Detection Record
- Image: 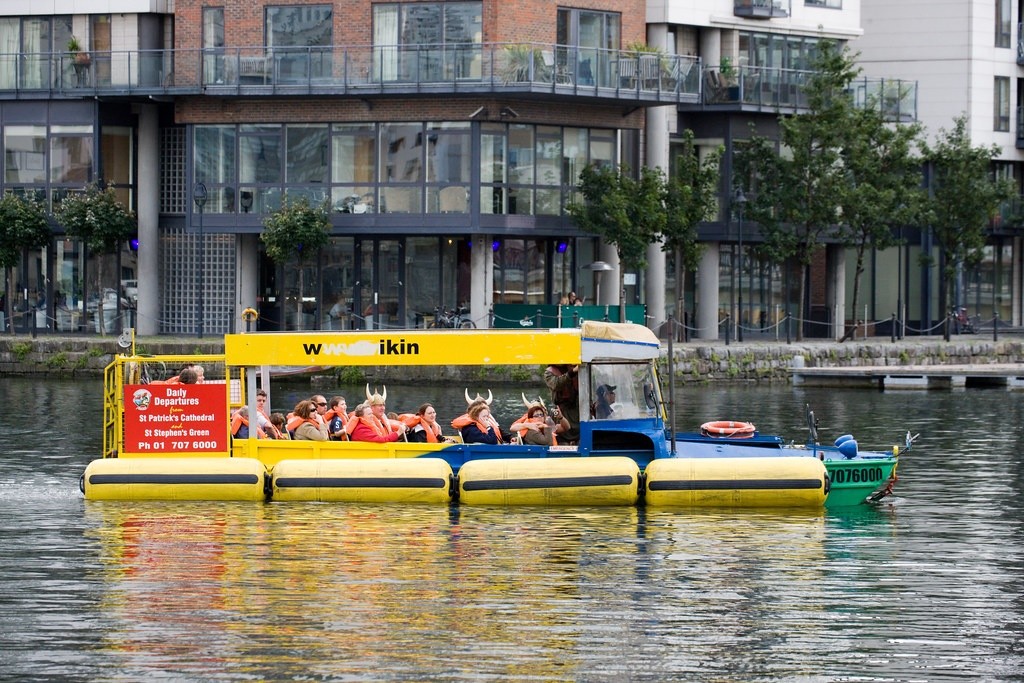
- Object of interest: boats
[79,309,923,515]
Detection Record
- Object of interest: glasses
[532,414,544,417]
[310,408,316,412]
[317,403,327,406]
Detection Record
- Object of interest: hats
[596,384,617,397]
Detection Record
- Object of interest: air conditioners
[224,55,272,77]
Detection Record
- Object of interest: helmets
[521,392,546,412]
[366,383,387,405]
[465,388,493,406]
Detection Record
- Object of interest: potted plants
[622,41,670,89]
[498,44,546,83]
[65,36,88,64]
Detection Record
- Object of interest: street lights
[732,189,749,343]
[580,261,615,306]
[192,182,207,339]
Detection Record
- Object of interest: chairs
[401,423,410,443]
[541,50,573,84]
[617,55,658,89]
[343,426,352,442]
[284,425,292,440]
[704,70,757,101]
[662,60,695,93]
[516,431,524,446]
[457,429,465,444]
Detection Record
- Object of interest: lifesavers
[701,420,755,433]
[700,432,755,439]
[241,308,258,322]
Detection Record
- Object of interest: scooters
[947,305,983,335]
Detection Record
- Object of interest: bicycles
[426,305,477,330]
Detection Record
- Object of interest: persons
[287,394,350,441]
[232,389,285,440]
[593,384,617,419]
[452,398,570,446]
[545,364,579,442]
[363,298,385,316]
[559,291,582,306]
[166,361,204,384]
[330,297,346,328]
[347,394,454,444]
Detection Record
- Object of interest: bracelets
[559,417,563,420]
